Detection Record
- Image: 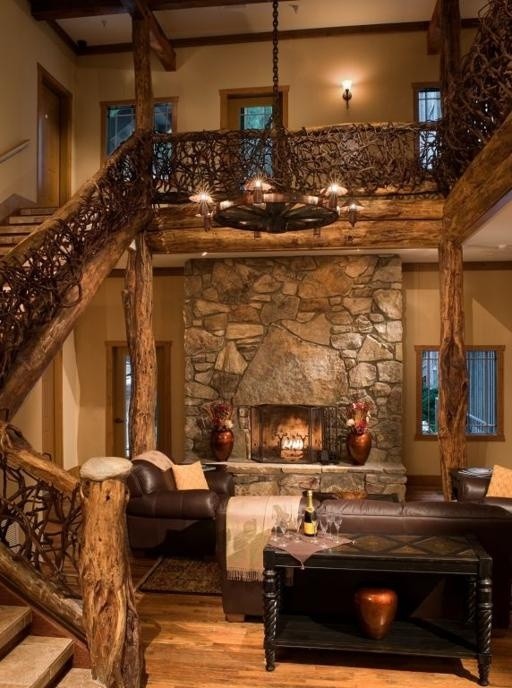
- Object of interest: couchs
[126,450,236,561]
[218,497,511,639]
[458,464,511,511]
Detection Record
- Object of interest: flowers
[204,404,234,431]
[343,401,371,434]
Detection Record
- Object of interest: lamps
[187,1,364,240]
[341,80,352,108]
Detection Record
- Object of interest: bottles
[302,489,319,537]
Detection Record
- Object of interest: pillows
[171,460,209,490]
[484,464,511,497]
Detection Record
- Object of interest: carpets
[133,558,225,596]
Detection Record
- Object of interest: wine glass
[310,509,343,546]
[267,510,306,548]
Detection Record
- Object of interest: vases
[346,433,372,466]
[210,430,235,462]
